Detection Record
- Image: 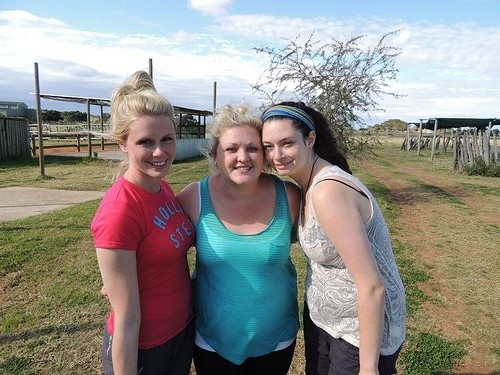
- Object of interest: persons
[258,101,410,375]
[88,67,200,374]
[99,101,305,375]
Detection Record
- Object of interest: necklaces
[299,154,319,228]
